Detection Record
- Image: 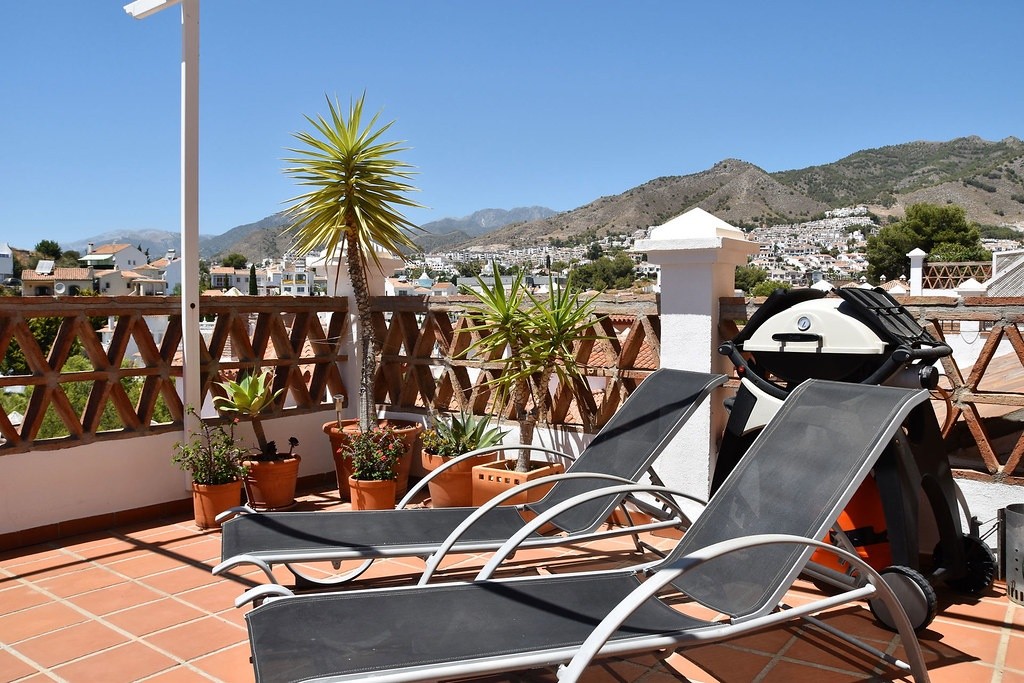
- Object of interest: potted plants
[210,364,301,508]
[420,408,511,507]
[169,402,246,528]
[446,257,617,533]
[278,89,425,500]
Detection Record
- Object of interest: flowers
[335,414,408,481]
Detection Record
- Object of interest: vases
[348,474,396,510]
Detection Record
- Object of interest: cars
[2,277,19,286]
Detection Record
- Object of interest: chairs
[212,368,730,600]
[247,376,926,683]
